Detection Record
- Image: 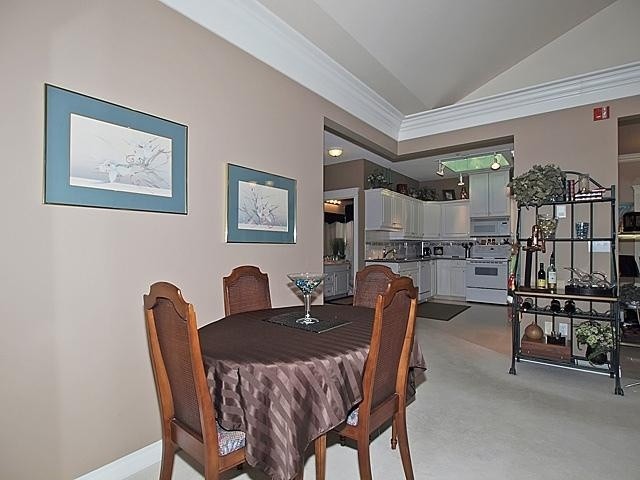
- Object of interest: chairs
[353,265,400,308]
[143,282,246,480]
[314,277,419,480]
[223,266,272,317]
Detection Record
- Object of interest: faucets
[383,248,396,258]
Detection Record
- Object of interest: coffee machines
[417,242,432,259]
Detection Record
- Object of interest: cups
[536,214,558,239]
[577,222,589,239]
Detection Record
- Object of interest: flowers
[575,322,616,359]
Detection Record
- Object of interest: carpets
[416,301,471,321]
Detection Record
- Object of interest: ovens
[464,260,509,305]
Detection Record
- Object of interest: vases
[586,344,607,365]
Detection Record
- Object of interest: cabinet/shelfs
[619,153,640,347]
[324,272,334,297]
[509,171,624,396]
[365,171,511,304]
[334,271,349,295]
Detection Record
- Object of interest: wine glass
[284,273,331,324]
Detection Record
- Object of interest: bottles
[551,300,561,315]
[522,297,533,312]
[546,256,557,289]
[465,245,470,258]
[564,302,576,317]
[536,262,546,290]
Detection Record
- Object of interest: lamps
[436,160,444,176]
[328,147,343,157]
[491,152,501,170]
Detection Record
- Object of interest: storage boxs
[521,334,571,363]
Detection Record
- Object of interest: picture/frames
[225,163,297,244]
[44,84,188,215]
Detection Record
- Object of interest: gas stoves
[465,256,509,262]
